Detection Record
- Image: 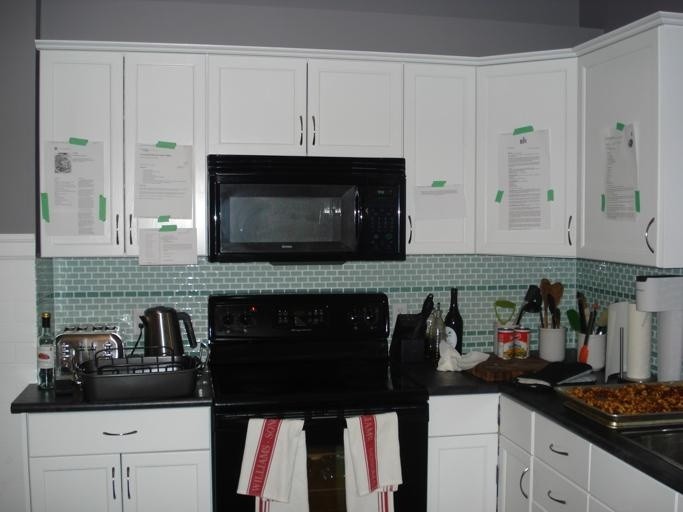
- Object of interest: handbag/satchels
[390,313,432,391]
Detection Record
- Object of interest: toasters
[53,325,126,374]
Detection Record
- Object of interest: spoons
[515,302,543,328]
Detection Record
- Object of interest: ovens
[213,403,427,512]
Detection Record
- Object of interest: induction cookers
[208,343,400,403]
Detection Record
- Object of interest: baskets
[95,344,183,375]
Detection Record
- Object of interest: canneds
[514,327,531,361]
[498,327,514,360]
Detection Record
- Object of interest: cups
[577,332,606,373]
[538,324,567,363]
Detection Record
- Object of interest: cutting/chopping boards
[469,357,548,382]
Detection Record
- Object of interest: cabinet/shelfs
[26,406,212,512]
[427,392,500,512]
[574,11,681,269]
[533,409,590,512]
[588,443,682,512]
[206,43,405,159]
[402,52,475,256]
[477,48,575,256]
[34,37,206,258]
[497,394,533,512]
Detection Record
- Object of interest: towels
[235,416,310,512]
[343,411,403,512]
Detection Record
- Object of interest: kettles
[138,306,198,356]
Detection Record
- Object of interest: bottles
[36,312,57,389]
[429,309,447,364]
[444,287,465,357]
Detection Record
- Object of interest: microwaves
[206,153,407,265]
